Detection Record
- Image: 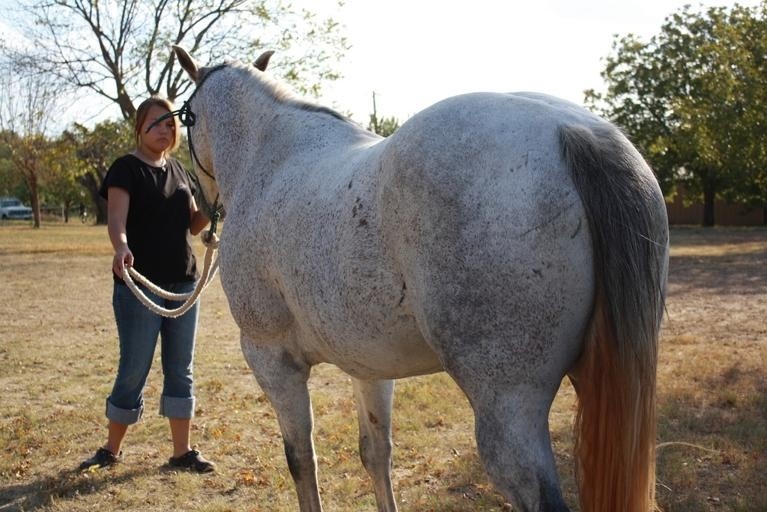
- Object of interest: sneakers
[168,444,216,472]
[80,447,122,470]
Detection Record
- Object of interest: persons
[78,97,222,474]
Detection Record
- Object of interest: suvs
[0,197,33,222]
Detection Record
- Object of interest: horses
[170,43,670,512]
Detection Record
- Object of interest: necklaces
[136,151,167,172]
